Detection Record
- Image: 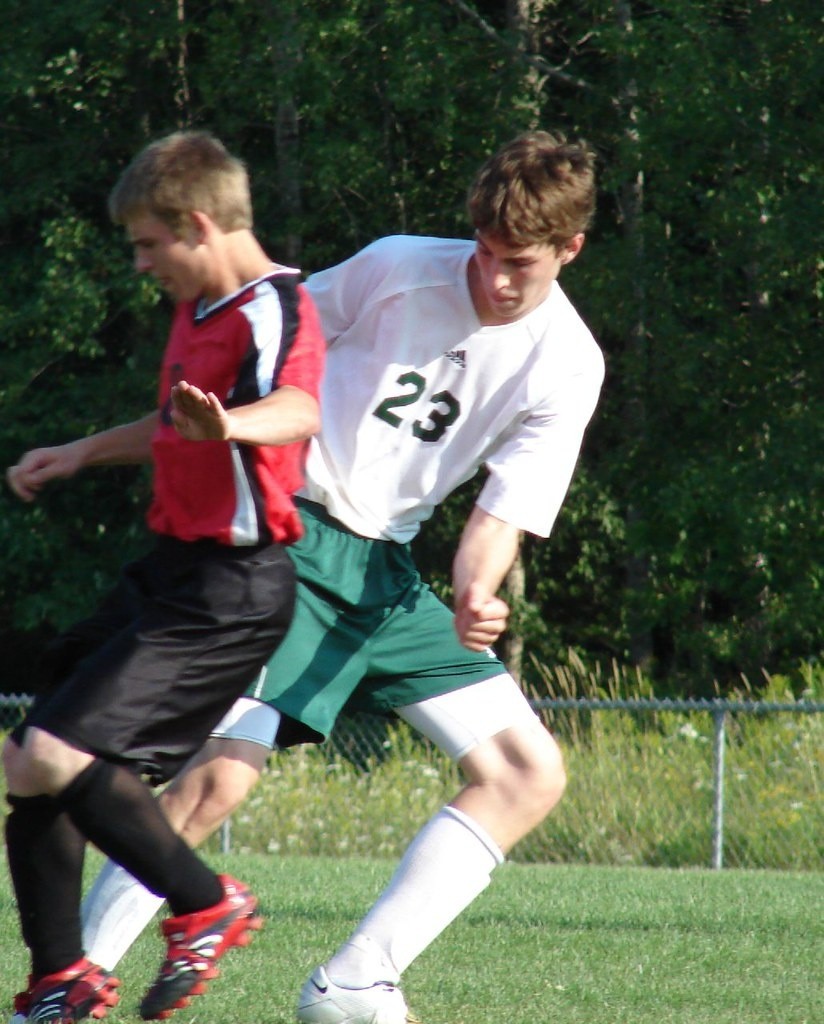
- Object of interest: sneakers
[12,957,121,1024]
[137,874,264,1020]
[297,965,420,1024]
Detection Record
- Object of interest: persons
[83,129,607,1023]
[3,130,324,1024]
